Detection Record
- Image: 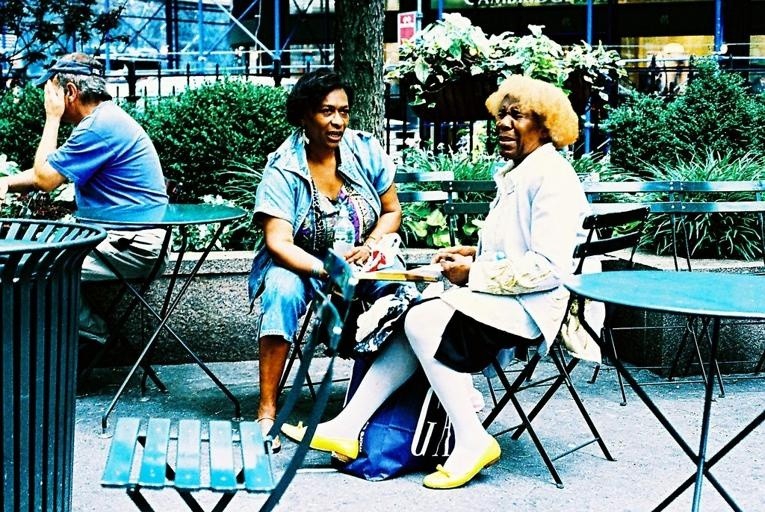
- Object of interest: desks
[562,269,765,512]
[71,202,249,439]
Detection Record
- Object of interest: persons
[0,52,173,389]
[281,73,610,490]
[247,69,403,455]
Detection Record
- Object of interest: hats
[31,60,105,89]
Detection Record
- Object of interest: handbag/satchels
[331,339,455,481]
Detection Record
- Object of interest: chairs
[100,249,344,510]
[47,180,184,395]
[439,178,498,242]
[581,180,715,407]
[394,168,454,266]
[675,179,765,394]
[484,207,649,487]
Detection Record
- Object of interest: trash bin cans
[0,217,107,512]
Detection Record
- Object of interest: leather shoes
[423,435,502,489]
[280,421,360,464]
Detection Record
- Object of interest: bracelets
[310,256,324,277]
[363,241,373,252]
